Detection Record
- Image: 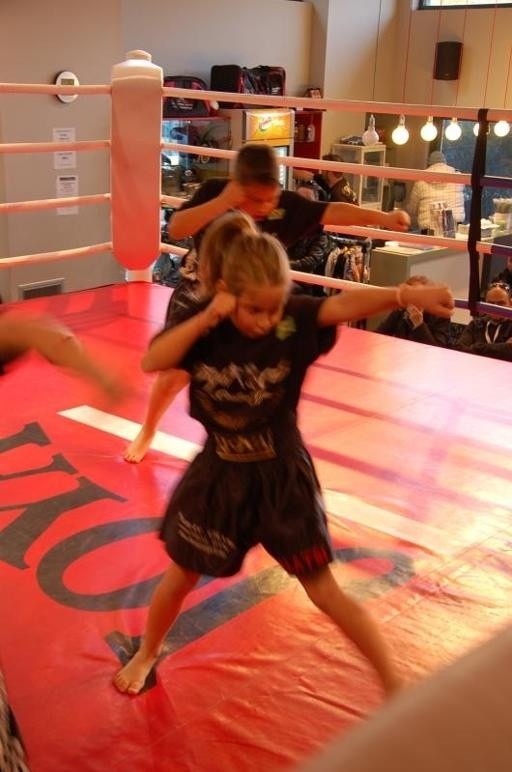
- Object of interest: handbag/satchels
[210,64,286,110]
[163,75,212,118]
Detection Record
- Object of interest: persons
[113,208,454,705]
[121,143,412,462]
[0,303,128,401]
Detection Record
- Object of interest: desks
[363,229,472,333]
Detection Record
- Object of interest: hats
[429,152,446,165]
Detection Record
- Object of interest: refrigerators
[217,107,295,193]
